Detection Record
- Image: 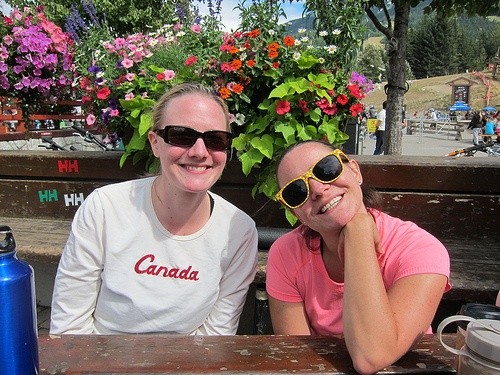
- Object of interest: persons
[358,100,500,155]
[50,84,259,335]
[266,139,452,375]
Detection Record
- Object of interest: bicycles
[443,140,500,157]
[38,126,123,150]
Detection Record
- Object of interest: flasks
[0,225,39,375]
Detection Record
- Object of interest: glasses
[153,124,234,152]
[274,148,350,212]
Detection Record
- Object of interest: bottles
[437,315,500,375]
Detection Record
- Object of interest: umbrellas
[482,106,496,115]
[448,101,471,114]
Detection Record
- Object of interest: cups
[453,303,500,372]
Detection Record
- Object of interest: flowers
[0,0,378,227]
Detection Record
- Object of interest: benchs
[478,133,500,143]
[0,149,500,337]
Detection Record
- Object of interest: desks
[35,333,465,375]
